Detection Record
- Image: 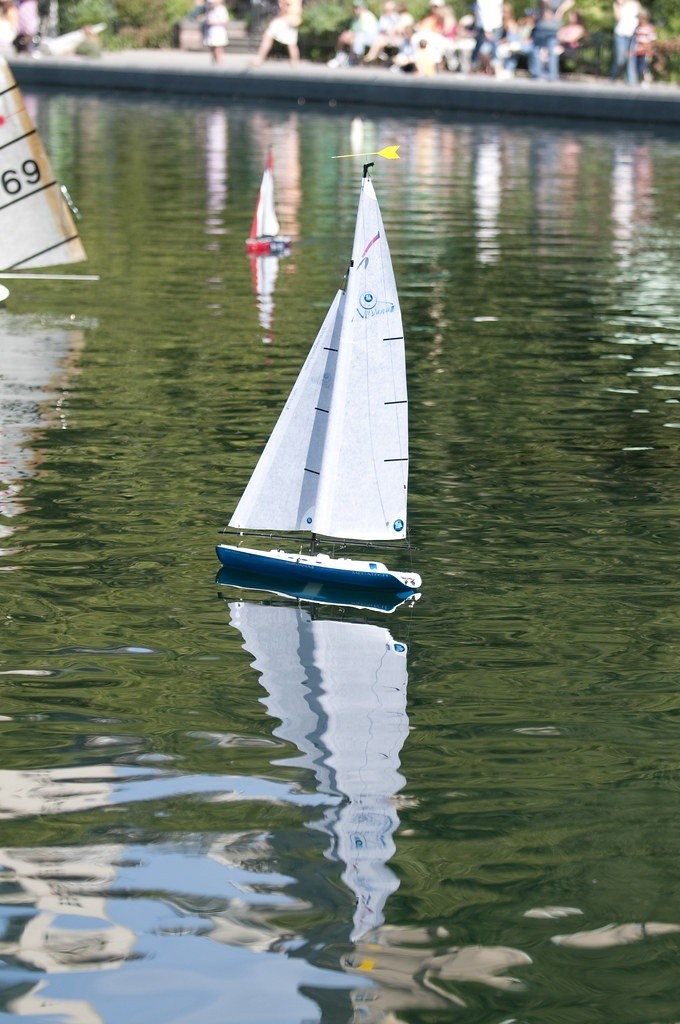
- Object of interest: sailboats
[0,53,100,303]
[244,146,291,255]
[208,566,426,977]
[208,143,424,593]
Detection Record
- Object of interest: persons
[0,0,102,59]
[191,0,659,87]
